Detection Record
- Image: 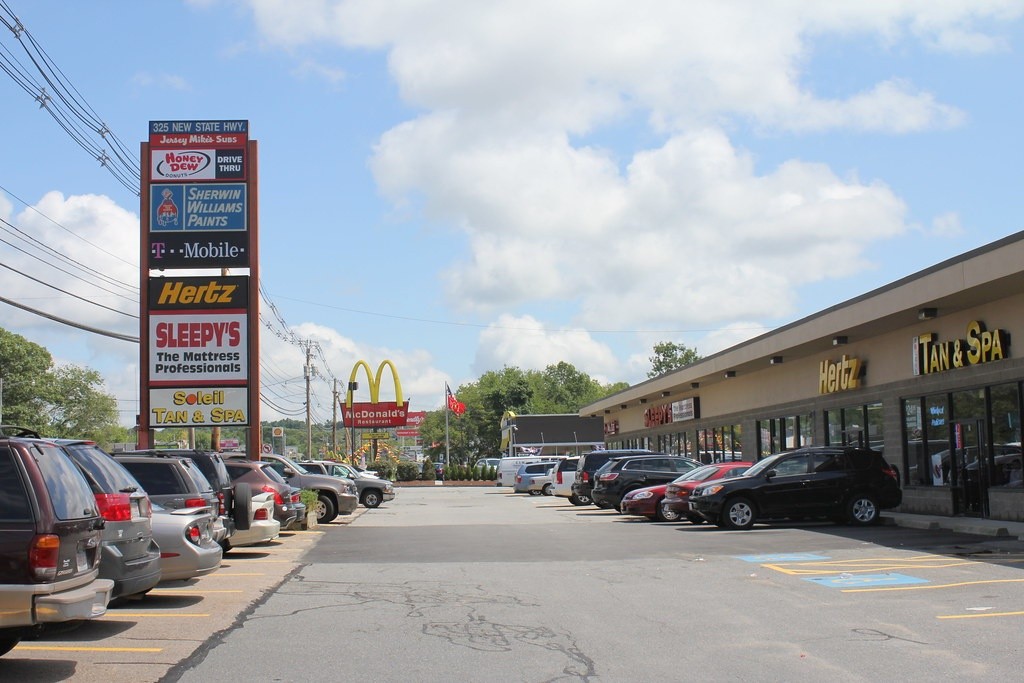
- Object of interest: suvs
[688,444,903,531]
[540,456,592,506]
[590,454,706,514]
[16,437,162,635]
[573,448,667,509]
[109,450,219,525]
[0,424,114,657]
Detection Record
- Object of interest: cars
[149,500,224,582]
[474,458,500,471]
[119,449,395,554]
[661,462,757,526]
[620,462,756,522]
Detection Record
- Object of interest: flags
[447,385,466,418]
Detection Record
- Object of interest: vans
[496,456,570,487]
[514,462,557,493]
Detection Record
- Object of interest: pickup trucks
[528,468,555,496]
